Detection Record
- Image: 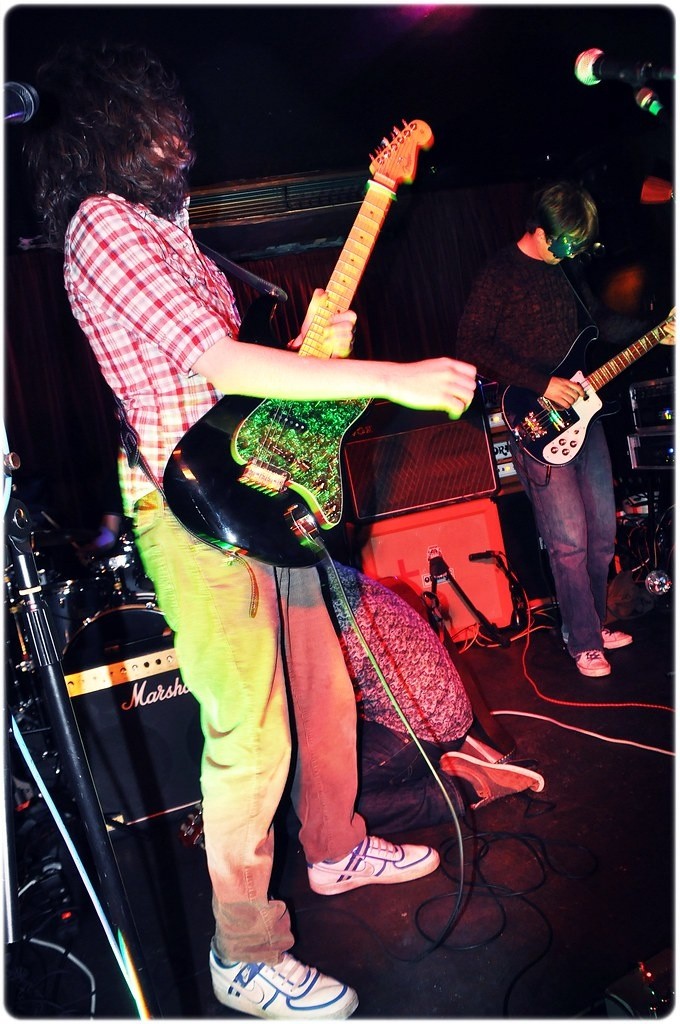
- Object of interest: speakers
[347,498,514,643]
[64,631,203,830]
[343,395,498,521]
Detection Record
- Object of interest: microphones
[574,47,675,86]
[636,88,674,126]
[4,82,38,124]
[469,551,502,561]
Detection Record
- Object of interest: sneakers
[210,936,358,1020]
[460,734,543,776]
[600,627,633,648]
[439,752,545,811]
[572,649,611,677]
[306,835,441,895]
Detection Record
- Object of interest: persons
[453,183,673,677]
[22,43,478,1020]
[278,560,544,852]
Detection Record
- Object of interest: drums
[57,592,212,879]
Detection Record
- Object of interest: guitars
[501,314,675,467]
[161,118,434,567]
